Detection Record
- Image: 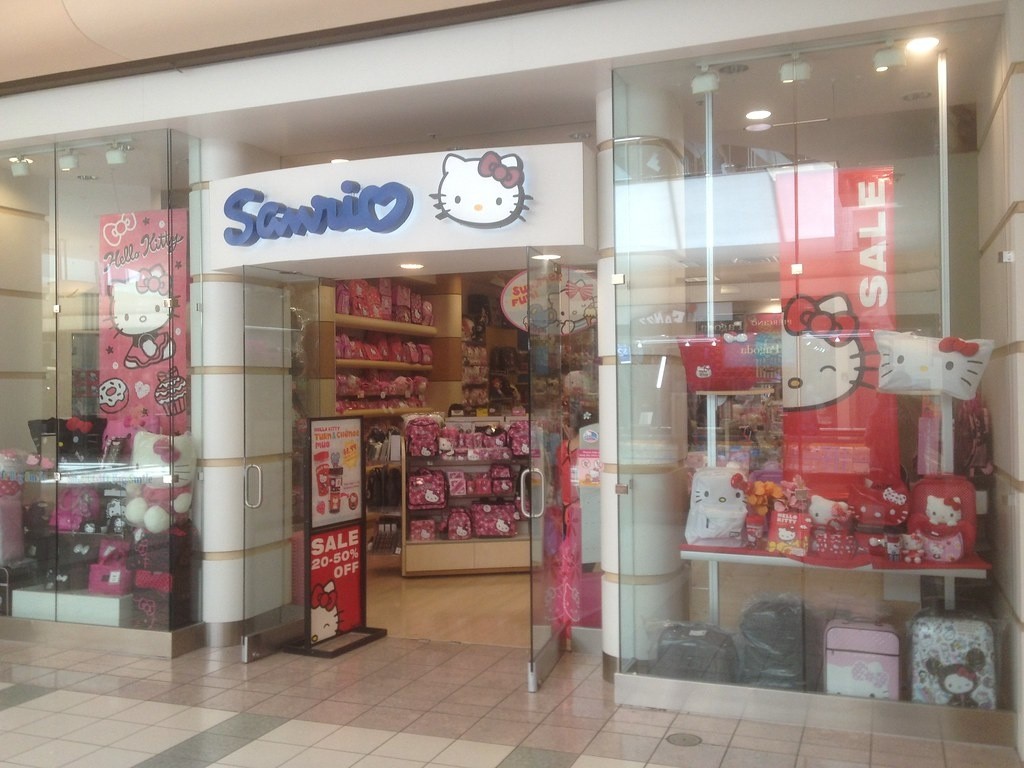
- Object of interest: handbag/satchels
[914,530,963,562]
[28,415,107,463]
[407,468,448,510]
[49,486,99,530]
[336,278,433,365]
[88,546,132,595]
[472,498,517,538]
[811,518,856,560]
[490,464,515,493]
[678,332,757,392]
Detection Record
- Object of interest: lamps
[60,149,86,171]
[690,65,719,96]
[106,142,134,164]
[872,39,906,72]
[10,156,33,178]
[778,51,810,82]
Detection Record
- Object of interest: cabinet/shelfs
[400,436,543,579]
[336,314,437,570]
[681,388,986,579]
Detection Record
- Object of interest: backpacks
[684,467,747,548]
[440,507,472,540]
[908,474,976,555]
[507,421,540,457]
[405,418,440,457]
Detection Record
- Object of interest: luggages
[912,608,998,710]
[658,627,736,684]
[823,617,903,701]
[740,602,816,691]
